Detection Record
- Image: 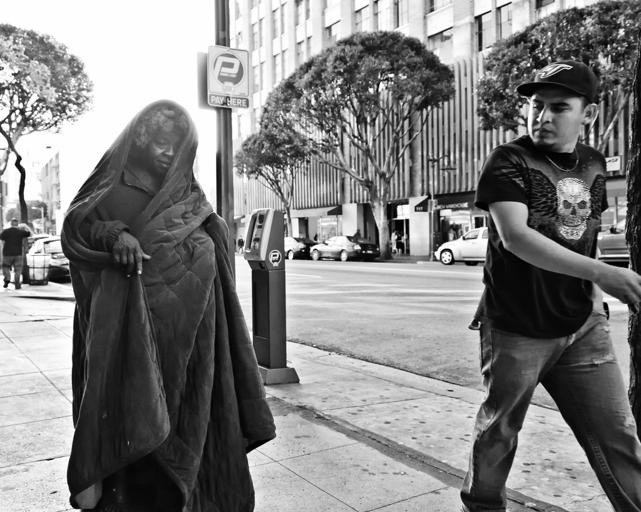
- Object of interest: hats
[516,59,598,101]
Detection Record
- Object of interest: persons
[400,232,408,254]
[459,58,641,512]
[354,229,362,240]
[0,218,31,290]
[391,229,400,254]
[58,99,277,512]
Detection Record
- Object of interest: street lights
[31,206,45,233]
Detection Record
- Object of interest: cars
[434,227,489,266]
[284,236,380,262]
[22,233,71,283]
[596,217,630,262]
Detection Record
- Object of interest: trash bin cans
[25,253,52,285]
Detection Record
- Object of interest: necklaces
[536,145,580,173]
